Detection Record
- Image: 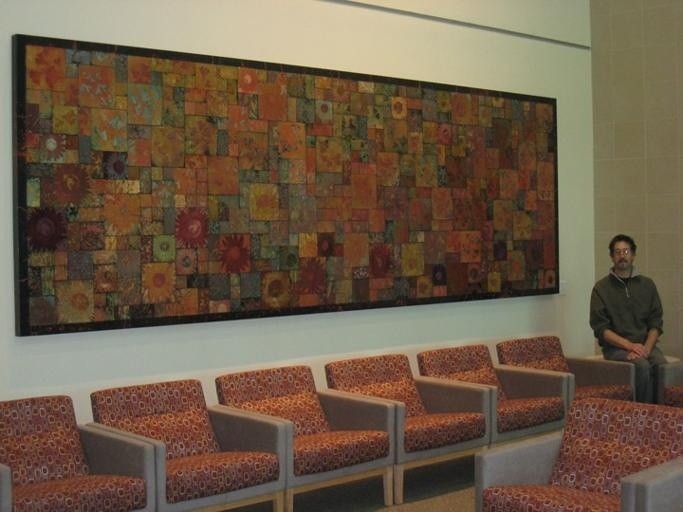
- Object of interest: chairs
[212,365,396,510]
[415,342,571,451]
[1,394,158,511]
[473,397,680,511]
[87,379,287,512]
[656,358,682,408]
[320,354,492,506]
[495,337,636,405]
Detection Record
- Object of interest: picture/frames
[12,31,562,336]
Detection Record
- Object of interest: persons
[590,234,667,406]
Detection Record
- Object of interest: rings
[638,352,640,355]
